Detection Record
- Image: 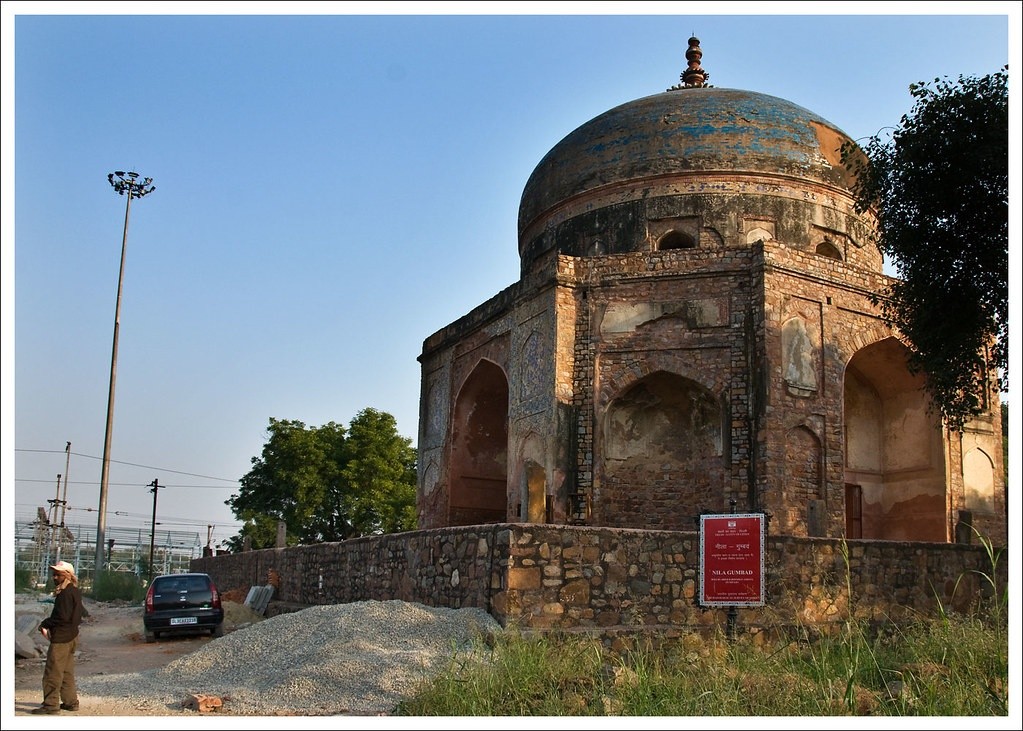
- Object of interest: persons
[31,560,89,715]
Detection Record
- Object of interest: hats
[49,562,73,574]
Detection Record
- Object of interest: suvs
[142,572,224,643]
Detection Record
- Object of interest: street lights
[90,166,157,594]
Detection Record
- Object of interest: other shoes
[60,701,79,711]
[31,708,61,715]
